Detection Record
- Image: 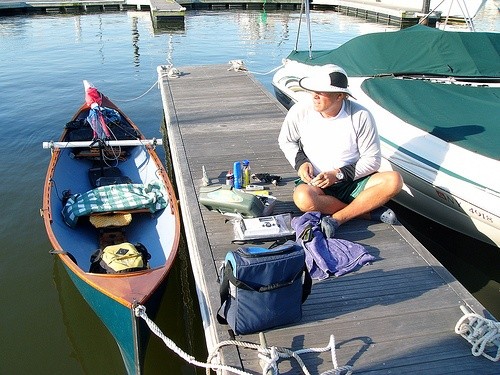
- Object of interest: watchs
[336,169,345,183]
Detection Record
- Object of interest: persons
[278,64,403,238]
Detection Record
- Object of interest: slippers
[321,215,339,239]
[370,203,397,225]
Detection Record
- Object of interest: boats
[40,80,181,364]
[269,22,500,250]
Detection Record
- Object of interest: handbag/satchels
[216,240,313,337]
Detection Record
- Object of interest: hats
[299,63,358,100]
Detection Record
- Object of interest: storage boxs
[198,184,277,219]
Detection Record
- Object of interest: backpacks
[90,242,152,274]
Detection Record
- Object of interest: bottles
[226,169,234,187]
[241,160,251,188]
